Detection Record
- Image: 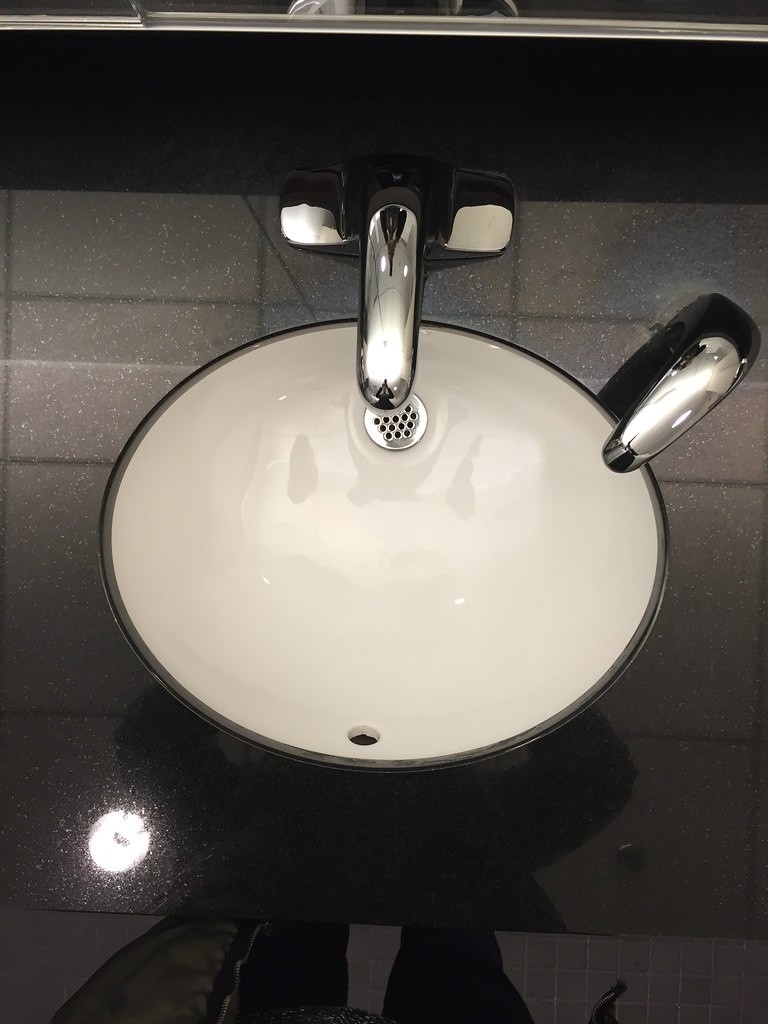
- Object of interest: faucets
[353,154,443,413]
[601,290,764,474]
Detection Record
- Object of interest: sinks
[97,315,672,778]
